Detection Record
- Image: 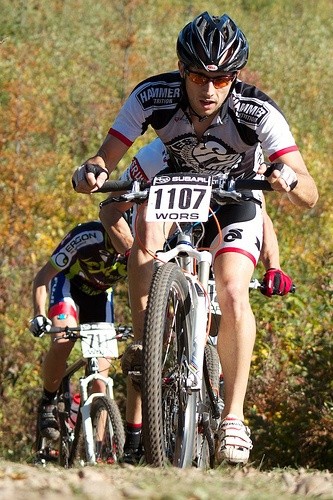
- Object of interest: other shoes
[122,428,143,463]
[215,418,253,465]
[121,341,145,391]
[37,396,61,439]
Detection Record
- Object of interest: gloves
[28,315,53,337]
[260,268,293,298]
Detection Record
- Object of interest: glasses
[183,67,237,89]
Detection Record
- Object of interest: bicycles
[93,174,297,473]
[33,324,127,471]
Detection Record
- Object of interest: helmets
[175,12,251,73]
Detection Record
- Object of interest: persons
[72,10,319,465]
[28,205,133,465]
